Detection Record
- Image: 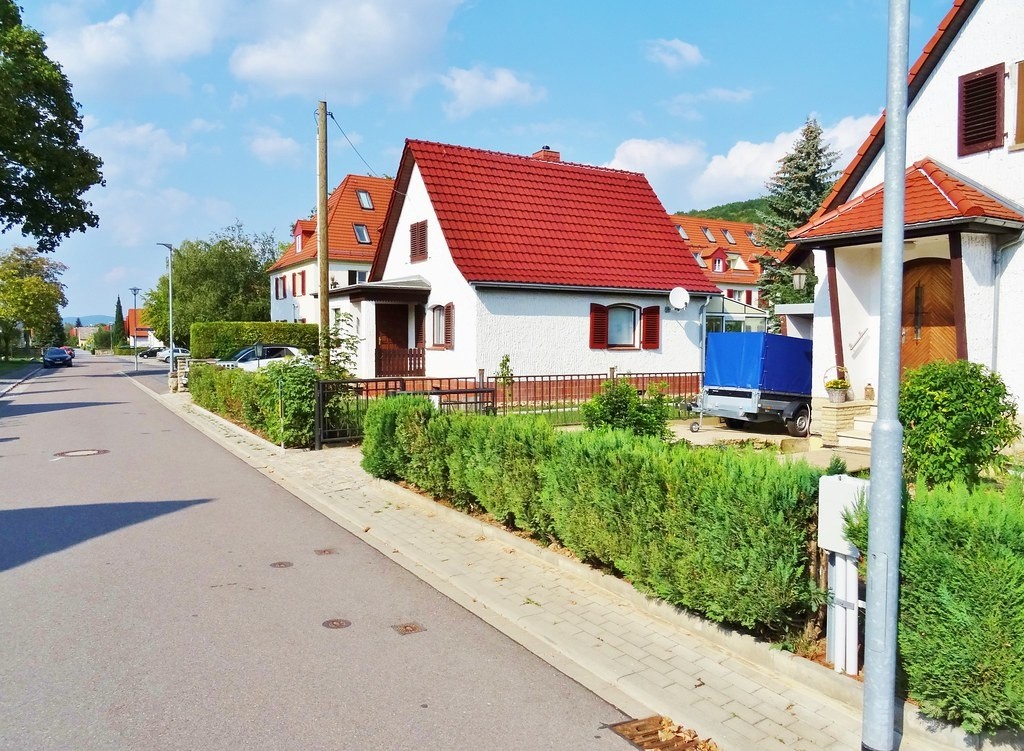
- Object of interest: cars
[43,346,75,368]
[139,347,167,359]
[182,345,316,388]
[157,348,190,362]
[129,287,142,371]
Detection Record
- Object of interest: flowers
[825,379,850,389]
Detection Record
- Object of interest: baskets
[823,365,851,403]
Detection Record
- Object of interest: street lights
[156,243,174,373]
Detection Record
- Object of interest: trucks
[688,331,813,437]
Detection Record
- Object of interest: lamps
[903,241,916,250]
